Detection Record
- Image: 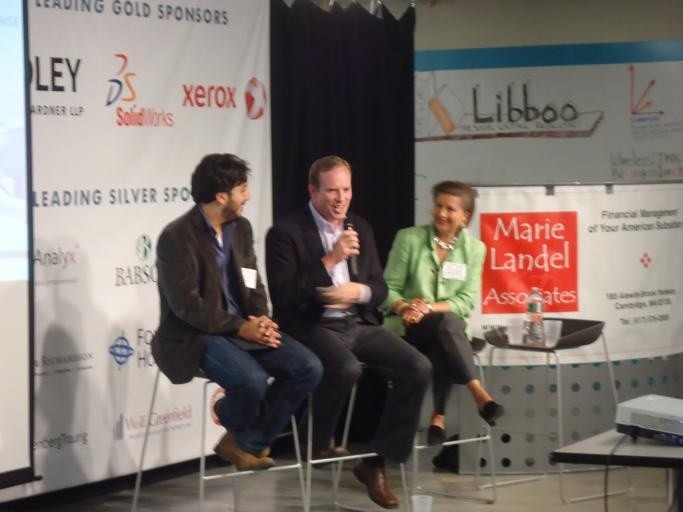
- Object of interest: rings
[411,316,416,321]
[412,302,415,307]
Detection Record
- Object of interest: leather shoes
[297,437,348,463]
[353,461,399,508]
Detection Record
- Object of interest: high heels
[429,424,445,446]
[478,401,504,426]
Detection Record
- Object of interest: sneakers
[212,398,276,470]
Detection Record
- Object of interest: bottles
[523,286,544,342]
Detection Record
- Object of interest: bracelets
[425,302,433,317]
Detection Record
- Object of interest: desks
[549,428,683,512]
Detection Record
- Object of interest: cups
[411,494,432,511]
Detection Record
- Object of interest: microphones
[342,217,359,280]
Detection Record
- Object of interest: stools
[130,359,306,512]
[474,318,627,501]
[330,334,500,510]
[231,357,410,512]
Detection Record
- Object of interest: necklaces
[431,231,458,251]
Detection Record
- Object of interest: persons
[373,179,504,446]
[152,153,324,472]
[265,156,432,508]
